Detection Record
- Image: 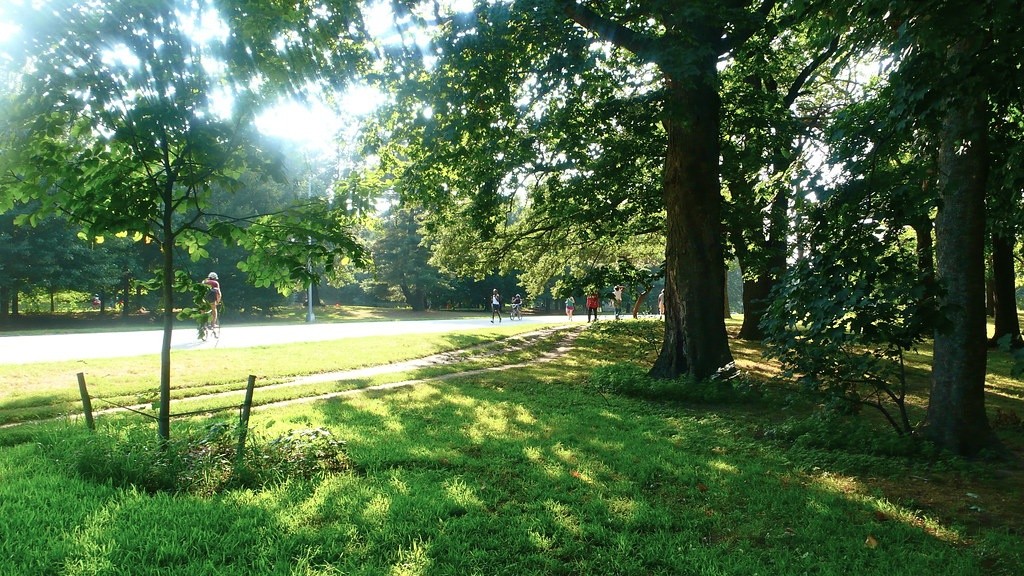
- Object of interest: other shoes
[491,321,494,323]
[499,318,501,323]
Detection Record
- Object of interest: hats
[516,294,520,297]
[209,272,219,280]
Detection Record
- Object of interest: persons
[658,288,665,320]
[512,294,523,320]
[198,272,222,328]
[490,288,503,324]
[612,285,626,321]
[586,291,600,323]
[565,294,576,322]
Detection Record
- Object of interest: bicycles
[509,302,523,320]
[198,301,223,341]
